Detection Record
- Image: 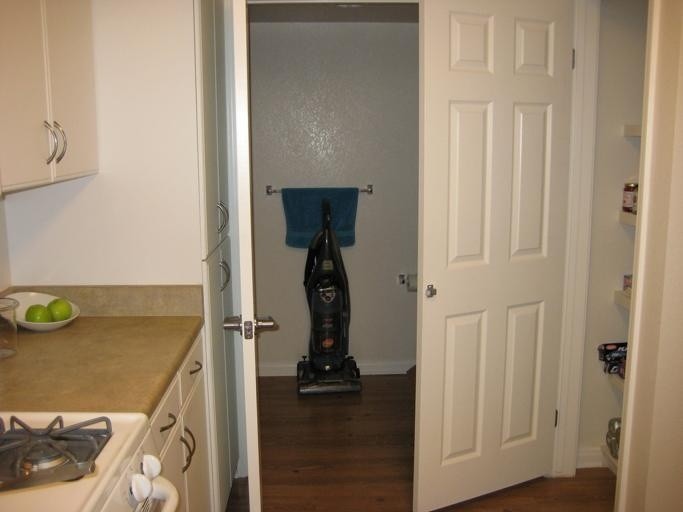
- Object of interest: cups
[0,298,20,360]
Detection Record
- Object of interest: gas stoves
[0,411,146,512]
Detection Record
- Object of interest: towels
[279,187,359,248]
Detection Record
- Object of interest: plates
[1,291,81,332]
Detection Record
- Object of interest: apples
[26,304,50,323]
[48,299,72,321]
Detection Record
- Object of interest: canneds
[623,183,637,212]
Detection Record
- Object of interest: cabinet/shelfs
[0,0,98,195]
[601,125,645,481]
[149,330,212,512]
[3,1,240,512]
